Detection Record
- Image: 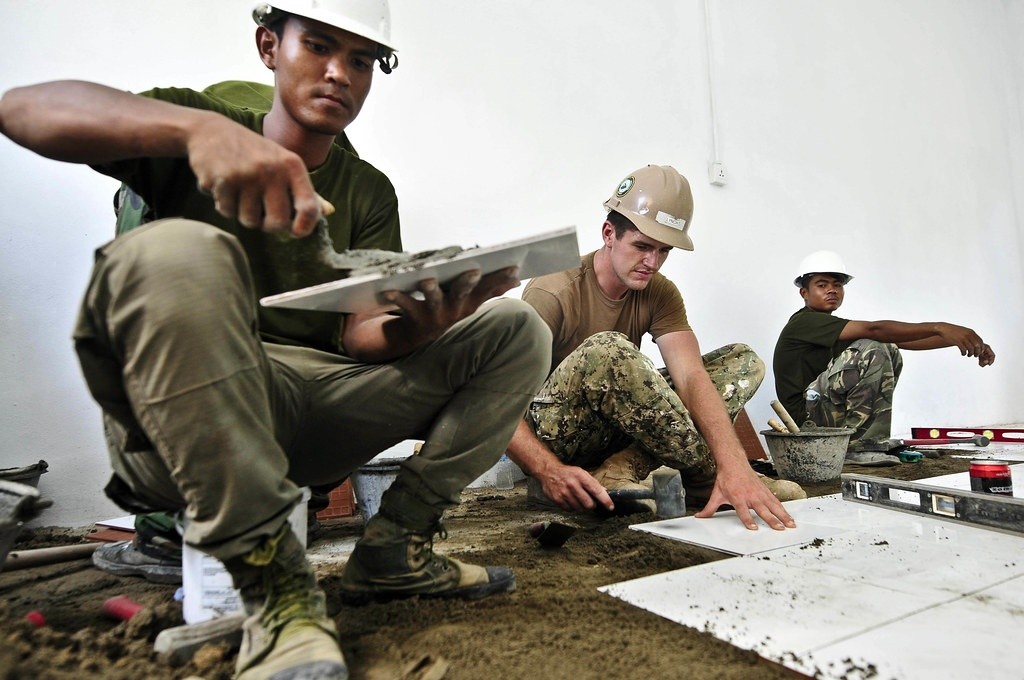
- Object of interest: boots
[586,442,657,516]
[233,524,349,680]
[682,453,807,506]
[340,514,517,601]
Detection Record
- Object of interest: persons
[504,165,797,530]
[92,511,182,584]
[0,0,553,680]
[774,251,996,465]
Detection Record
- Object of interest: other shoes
[94,533,185,585]
[842,451,902,466]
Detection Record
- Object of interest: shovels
[194,183,408,270]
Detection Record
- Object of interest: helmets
[792,250,855,287]
[602,164,694,252]
[264,0,400,51]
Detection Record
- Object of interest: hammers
[904,433,990,447]
[589,468,686,519]
[105,594,247,662]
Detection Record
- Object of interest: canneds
[969,460,1013,497]
